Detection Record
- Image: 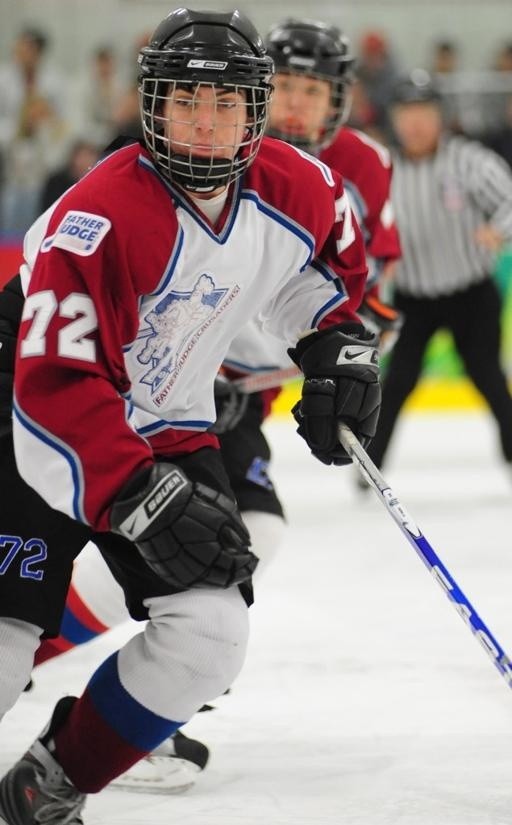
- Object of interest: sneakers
[0,740,85,825]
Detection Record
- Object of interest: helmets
[264,16,359,155]
[137,7,275,198]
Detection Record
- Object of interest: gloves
[287,319,383,466]
[108,460,259,592]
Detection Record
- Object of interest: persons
[0,6,386,825]
[20,17,409,774]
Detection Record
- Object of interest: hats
[388,80,437,105]
